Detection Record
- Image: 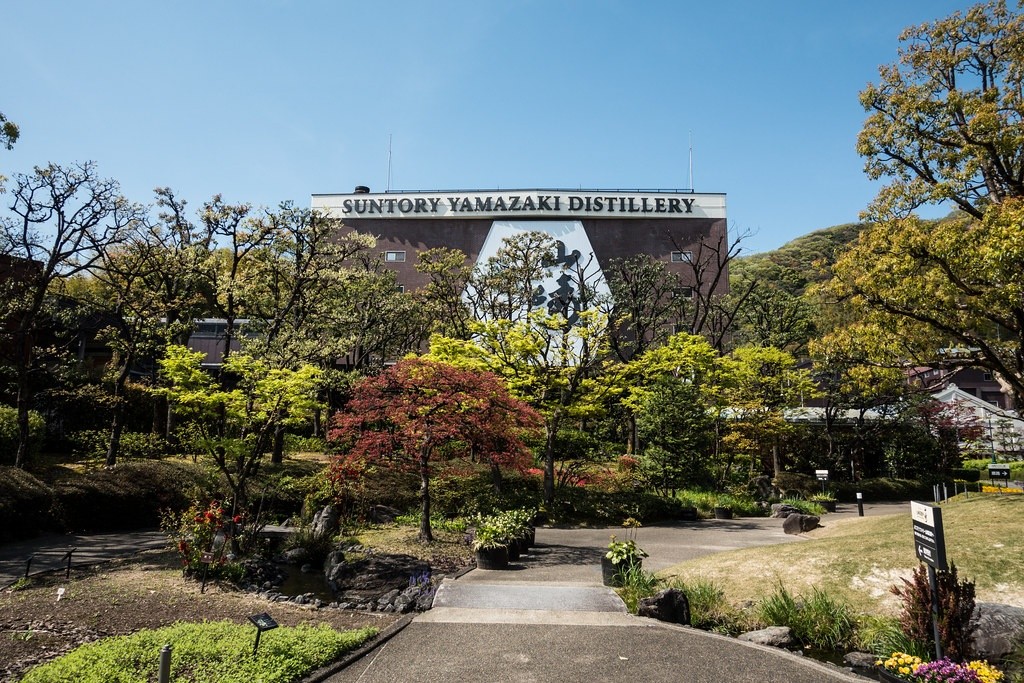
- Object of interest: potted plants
[469,506,537,570]
[601,517,649,587]
[810,491,837,513]
[713,502,734,520]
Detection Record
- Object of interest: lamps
[856,493,863,499]
